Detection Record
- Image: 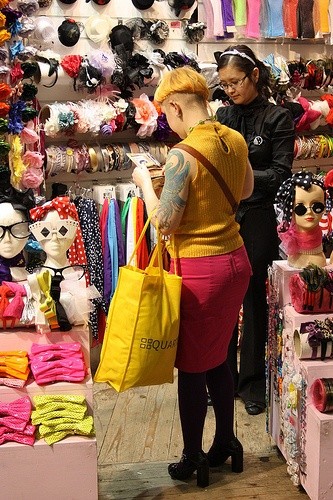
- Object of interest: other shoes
[245,401,266,414]
[207,392,212,405]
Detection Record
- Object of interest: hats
[108,25,132,51]
[57,19,79,46]
[28,17,56,50]
[84,14,112,42]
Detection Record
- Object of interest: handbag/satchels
[94,209,181,392]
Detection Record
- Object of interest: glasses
[293,202,326,215]
[0,221,34,239]
[219,73,250,90]
[32,265,90,281]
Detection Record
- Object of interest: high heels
[206,437,243,472]
[168,449,210,487]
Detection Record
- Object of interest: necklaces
[185,116,214,138]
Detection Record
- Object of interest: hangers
[62,178,92,200]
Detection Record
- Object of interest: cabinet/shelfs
[268,259,332,500]
[0,279,99,500]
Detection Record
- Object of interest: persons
[208,45,296,415]
[132,67,254,488]
[275,169,333,268]
[0,198,79,282]
